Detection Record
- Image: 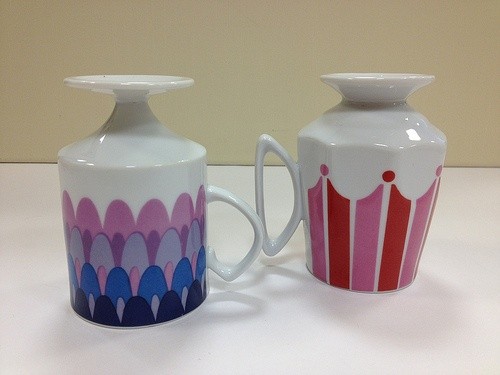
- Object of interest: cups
[252,71,448,294]
[56,73,262,329]
